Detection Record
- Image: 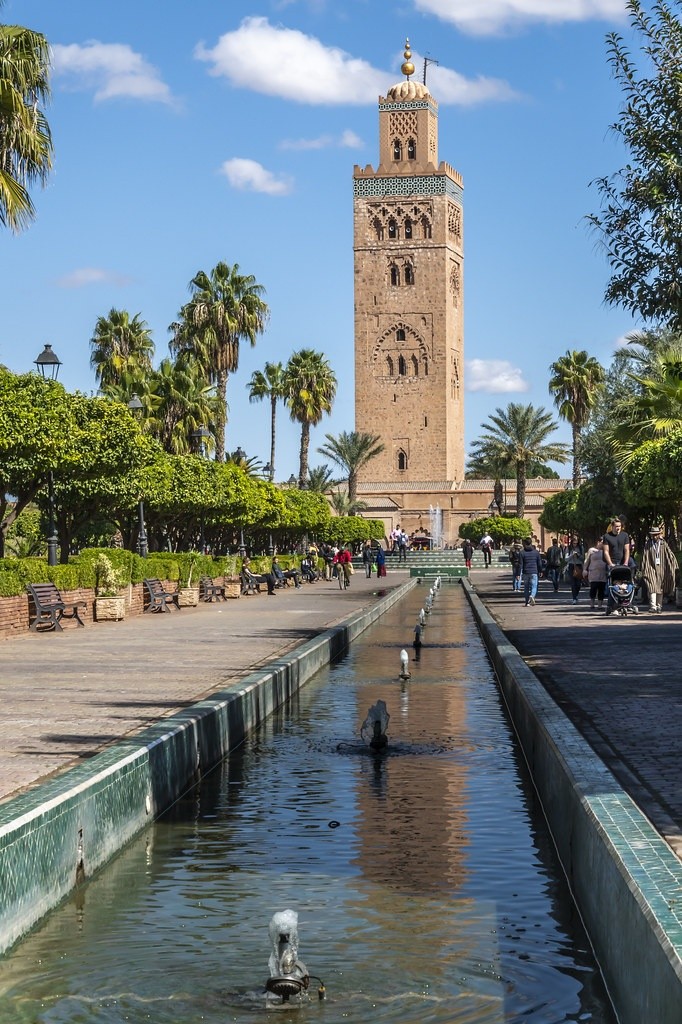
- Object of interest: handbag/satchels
[380,564,386,576]
[572,566,583,580]
[371,562,377,572]
[344,562,355,577]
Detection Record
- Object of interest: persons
[565,537,584,605]
[510,539,522,591]
[462,539,473,569]
[272,557,303,589]
[559,539,566,556]
[614,580,628,594]
[603,518,630,616]
[389,525,401,556]
[629,557,649,605]
[398,529,408,563]
[242,556,276,595]
[363,544,385,578]
[411,527,428,537]
[480,531,494,569]
[333,547,351,586]
[582,537,606,610]
[546,538,563,593]
[516,538,543,607]
[292,541,340,584]
[640,526,679,613]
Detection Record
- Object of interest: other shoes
[657,609,661,614]
[588,606,594,611]
[346,582,349,586]
[529,596,535,606]
[572,600,577,604]
[525,604,530,607]
[649,609,656,613]
[295,584,303,589]
[268,592,276,595]
[607,605,619,616]
[598,604,604,610]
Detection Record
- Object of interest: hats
[649,527,661,534]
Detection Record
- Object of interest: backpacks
[399,534,408,546]
[511,548,521,567]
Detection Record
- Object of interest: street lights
[563,480,573,548]
[190,422,212,558]
[231,447,248,557]
[313,534,314,541]
[261,462,276,557]
[316,534,317,543]
[287,474,299,554]
[127,392,148,560]
[31,343,64,565]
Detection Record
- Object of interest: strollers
[604,566,641,617]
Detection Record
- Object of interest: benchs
[199,576,228,603]
[271,568,290,590]
[239,572,264,596]
[144,576,182,613]
[28,583,87,634]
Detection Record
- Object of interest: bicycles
[335,562,350,589]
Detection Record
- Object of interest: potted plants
[178,551,201,608]
[225,553,241,598]
[259,557,270,590]
[91,553,126,621]
